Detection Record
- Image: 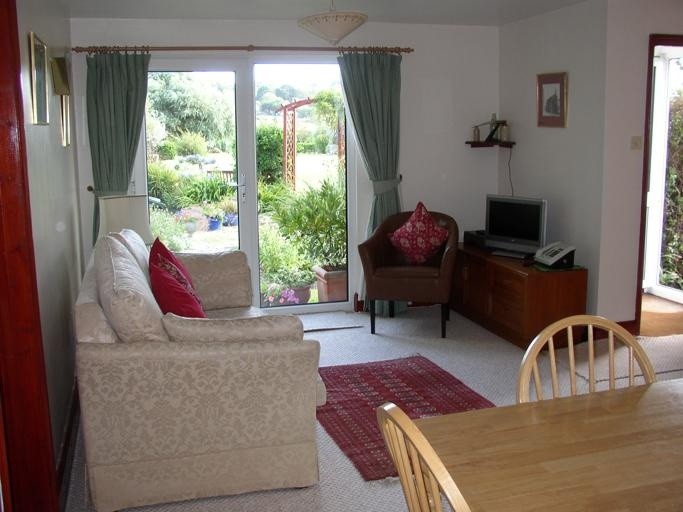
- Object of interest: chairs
[516,313,659,405]
[374,398,473,512]
[356,211,460,341]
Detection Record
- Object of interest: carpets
[315,349,499,484]
[542,335,683,383]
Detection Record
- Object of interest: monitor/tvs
[484,194,547,259]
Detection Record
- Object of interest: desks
[403,378,683,510]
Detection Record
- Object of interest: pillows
[384,199,449,266]
[148,237,208,321]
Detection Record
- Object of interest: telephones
[534,241,576,270]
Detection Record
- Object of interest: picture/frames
[27,27,52,127]
[532,70,566,130]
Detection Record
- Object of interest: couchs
[66,232,329,512]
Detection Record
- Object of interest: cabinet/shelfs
[455,242,589,354]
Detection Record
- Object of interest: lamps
[296,0,369,45]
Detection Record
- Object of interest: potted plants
[175,183,346,308]
